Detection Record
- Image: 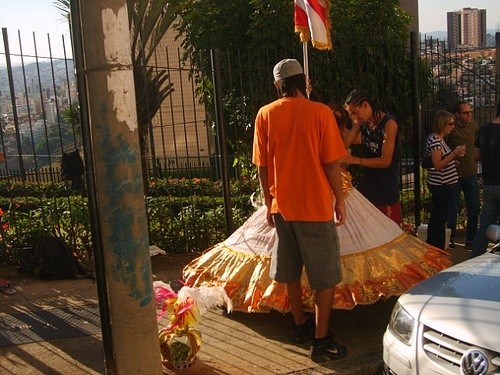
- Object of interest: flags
[293,0,332,51]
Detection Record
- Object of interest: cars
[381,222,500,375]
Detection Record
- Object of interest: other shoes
[466,242,473,250]
[309,339,348,362]
[291,319,334,344]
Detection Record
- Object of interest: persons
[472,101,500,258]
[328,84,402,227]
[423,110,465,250]
[251,58,348,364]
[443,101,481,247]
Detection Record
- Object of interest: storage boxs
[418,223,452,250]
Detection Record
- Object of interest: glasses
[459,111,473,114]
[448,122,455,126]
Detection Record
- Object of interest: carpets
[0,304,100,347]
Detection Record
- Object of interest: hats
[272,58,304,82]
